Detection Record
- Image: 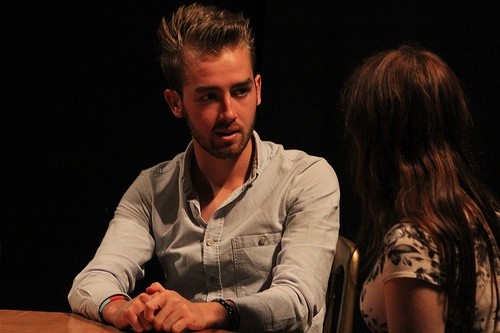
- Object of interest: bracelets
[212,298,234,329]
[99,295,130,318]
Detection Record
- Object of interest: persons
[347,48,500,333]
[67,4,341,333]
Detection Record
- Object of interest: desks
[0,307,123,333]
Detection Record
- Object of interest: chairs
[324,236,361,333]
